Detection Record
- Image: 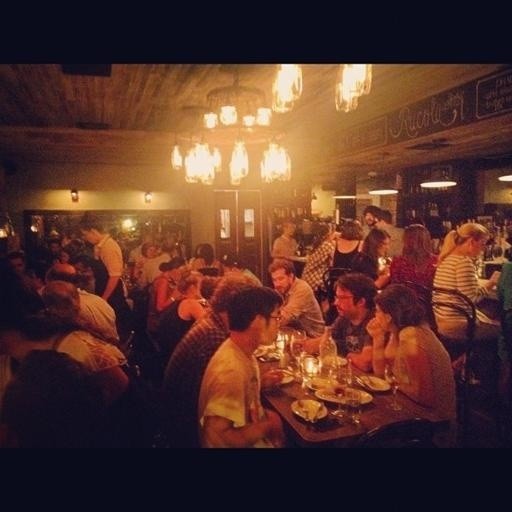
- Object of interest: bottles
[316,326,339,380]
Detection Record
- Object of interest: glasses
[335,295,352,299]
[272,317,281,321]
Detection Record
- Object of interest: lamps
[169,62,307,187]
[331,63,372,112]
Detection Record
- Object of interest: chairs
[325,267,357,320]
[408,280,485,433]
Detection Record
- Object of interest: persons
[199,285,291,446]
[355,285,459,429]
[129,236,224,367]
[269,205,441,315]
[266,259,324,341]
[4,237,121,344]
[289,273,378,372]
[431,222,502,388]
[78,210,132,323]
[5,264,150,447]
[161,267,262,422]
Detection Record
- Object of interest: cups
[302,357,320,382]
[346,391,360,424]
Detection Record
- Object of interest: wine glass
[330,360,352,416]
[290,331,305,376]
[384,354,405,412]
[275,332,288,369]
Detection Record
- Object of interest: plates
[292,401,326,421]
[315,387,374,405]
[267,370,294,384]
[361,376,390,393]
